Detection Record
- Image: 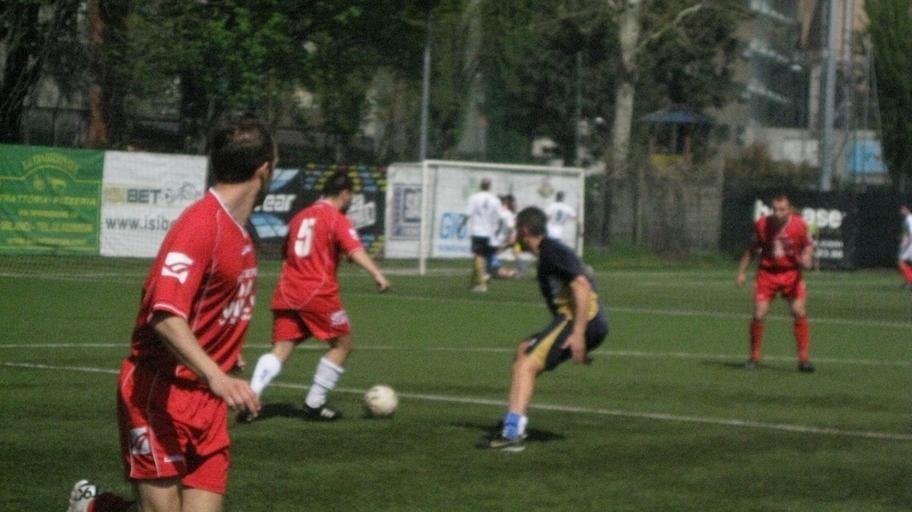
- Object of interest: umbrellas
[637,102,713,151]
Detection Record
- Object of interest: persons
[66,129,279,512]
[896,200,912,286]
[465,176,504,295]
[497,191,527,278]
[736,188,817,371]
[540,186,581,237]
[474,204,610,454]
[237,169,392,422]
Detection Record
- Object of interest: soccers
[365,386,398,417]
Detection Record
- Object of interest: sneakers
[64,477,104,511]
[301,402,342,420]
[799,361,815,371]
[237,410,254,424]
[479,432,529,454]
